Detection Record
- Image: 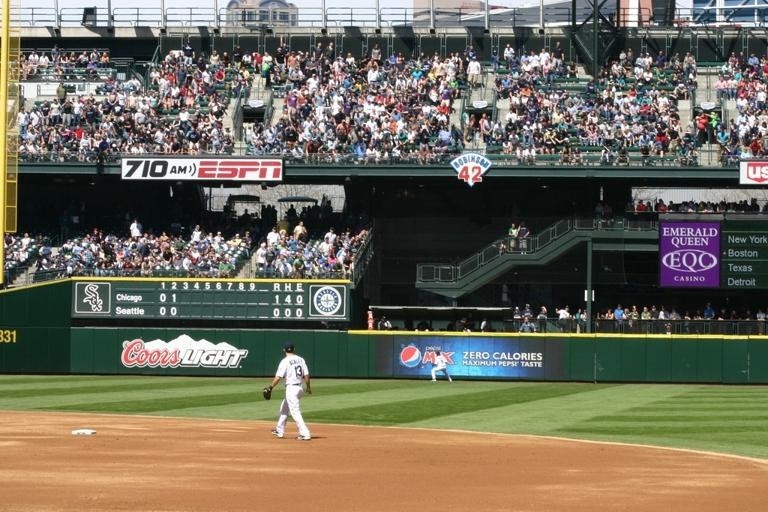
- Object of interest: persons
[456,317,472,332]
[501,221,529,255]
[431,351,452,382]
[593,197,767,232]
[5,41,768,168]
[417,319,429,330]
[4,192,372,280]
[264,344,312,440]
[378,316,392,330]
[511,302,766,335]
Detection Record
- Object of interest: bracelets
[268,385,273,390]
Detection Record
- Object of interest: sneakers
[295,435,311,441]
[271,430,284,438]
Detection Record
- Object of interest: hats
[286,342,295,347]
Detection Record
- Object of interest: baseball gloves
[263,387,271,400]
